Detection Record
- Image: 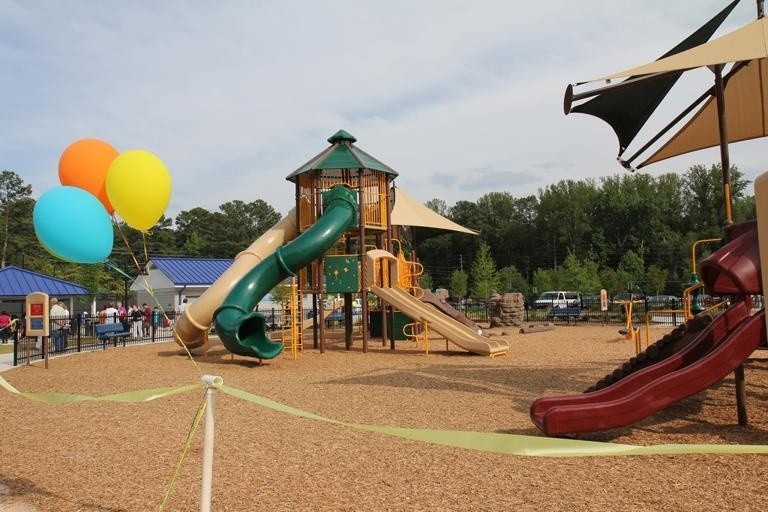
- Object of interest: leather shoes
[51,351,64,354]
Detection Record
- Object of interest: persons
[0,311,12,343]
[99,302,158,338]
[285,306,291,325]
[179,299,188,314]
[50,298,69,353]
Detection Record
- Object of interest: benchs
[94,322,132,350]
[549,308,580,322]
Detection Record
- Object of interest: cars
[352,298,362,314]
[581,290,764,313]
[447,295,486,310]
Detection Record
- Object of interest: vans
[534,289,580,311]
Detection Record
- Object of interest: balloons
[105,149,171,232]
[33,186,114,264]
[59,138,121,214]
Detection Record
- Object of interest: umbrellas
[564,0,768,426]
[365,181,480,240]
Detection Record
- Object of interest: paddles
[174,208,298,348]
[371,286,509,353]
[529,297,764,437]
[212,184,357,359]
